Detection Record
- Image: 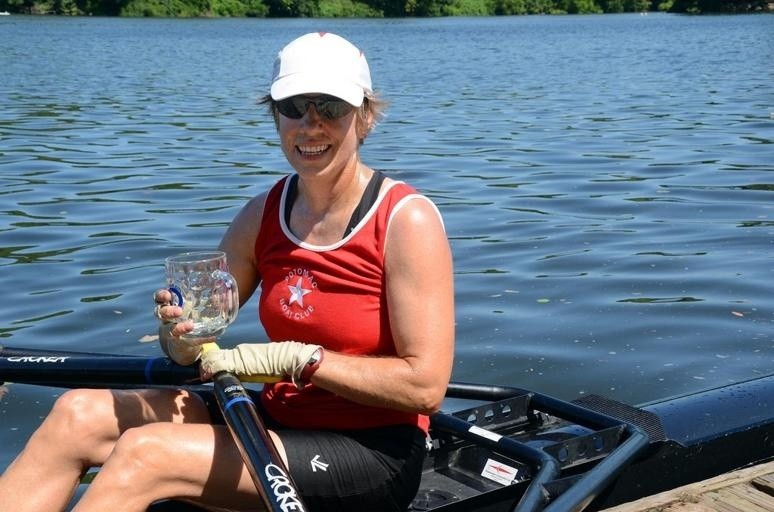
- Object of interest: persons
[0,33,456,512]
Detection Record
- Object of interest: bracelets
[300,348,324,382]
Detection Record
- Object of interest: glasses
[272,94,354,120]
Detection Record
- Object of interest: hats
[271,30,373,109]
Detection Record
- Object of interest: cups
[161,246,241,345]
